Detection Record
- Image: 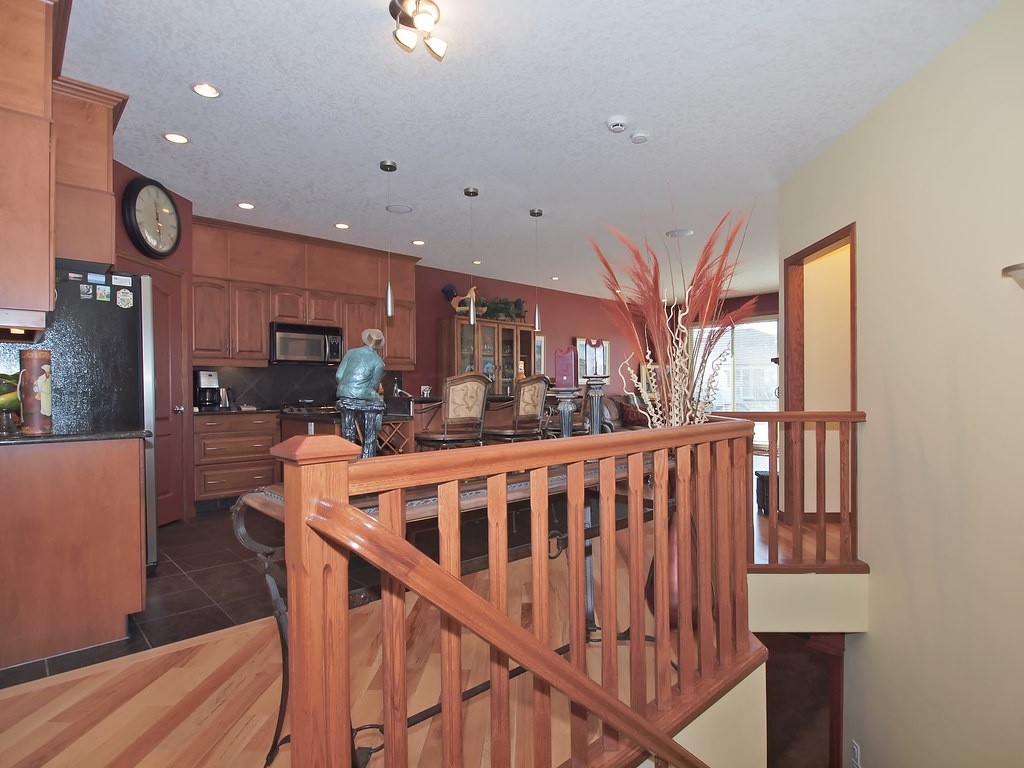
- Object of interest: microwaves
[269,322,343,367]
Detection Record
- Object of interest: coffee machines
[193,370,220,412]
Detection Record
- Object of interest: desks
[229,451,691,768]
[413,394,515,404]
[755,471,780,515]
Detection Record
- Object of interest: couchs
[596,395,697,444]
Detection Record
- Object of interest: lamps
[380,159,396,318]
[385,0,448,58]
[529,208,543,332]
[464,187,479,326]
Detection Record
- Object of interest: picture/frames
[639,363,682,400]
[536,335,546,376]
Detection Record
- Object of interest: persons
[336,327,387,450]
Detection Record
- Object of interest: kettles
[219,387,229,408]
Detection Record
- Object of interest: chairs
[487,374,552,443]
[412,372,494,449]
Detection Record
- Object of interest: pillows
[621,402,654,426]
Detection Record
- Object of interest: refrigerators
[40,268,158,579]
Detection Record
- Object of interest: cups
[19,349,53,436]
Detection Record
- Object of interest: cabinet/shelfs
[0,0,537,669]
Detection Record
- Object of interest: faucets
[395,387,413,397]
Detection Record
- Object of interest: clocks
[122,177,182,259]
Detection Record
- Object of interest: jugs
[200,390,220,405]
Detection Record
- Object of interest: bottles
[393,377,399,397]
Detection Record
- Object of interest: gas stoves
[280,401,341,418]
